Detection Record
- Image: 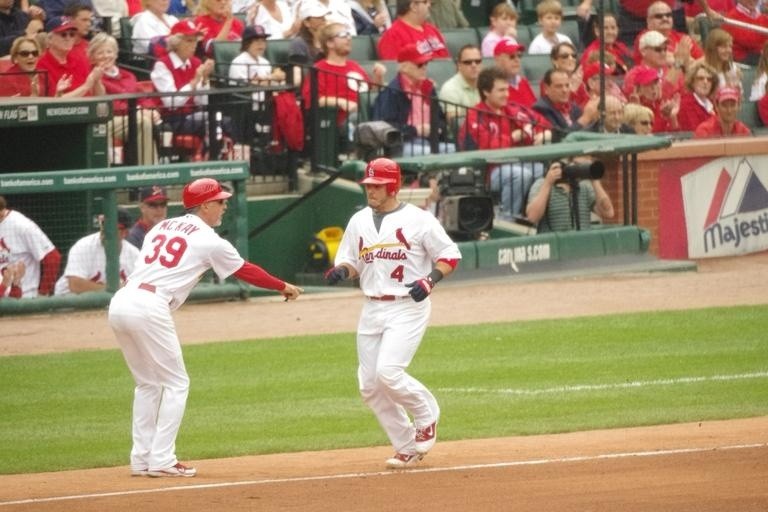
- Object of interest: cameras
[555,159,604,182]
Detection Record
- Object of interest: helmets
[182,178,233,210]
[359,157,402,197]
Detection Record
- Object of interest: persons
[107,178,305,479]
[323,157,463,469]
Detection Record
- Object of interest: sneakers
[415,420,437,454]
[386,453,425,468]
[131,469,148,476]
[148,463,196,477]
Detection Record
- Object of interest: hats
[396,46,433,65]
[141,185,171,205]
[581,61,614,83]
[241,26,272,41]
[493,40,525,57]
[297,0,332,22]
[715,87,740,105]
[98,210,133,229]
[631,66,658,86]
[638,30,670,51]
[171,20,203,36]
[46,16,78,34]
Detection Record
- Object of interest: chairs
[212,40,241,76]
[739,101,762,127]
[266,39,293,69]
[439,29,479,57]
[350,34,374,60]
[361,60,398,84]
[522,55,553,80]
[427,60,457,87]
[528,21,580,47]
[740,70,759,101]
[478,24,531,46]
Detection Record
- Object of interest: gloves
[403,277,434,302]
[323,264,349,286]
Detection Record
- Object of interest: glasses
[13,49,39,60]
[462,60,482,66]
[635,121,653,127]
[557,52,577,59]
[649,13,674,20]
[330,32,352,40]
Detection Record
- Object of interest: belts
[366,294,410,301]
[123,280,174,307]
